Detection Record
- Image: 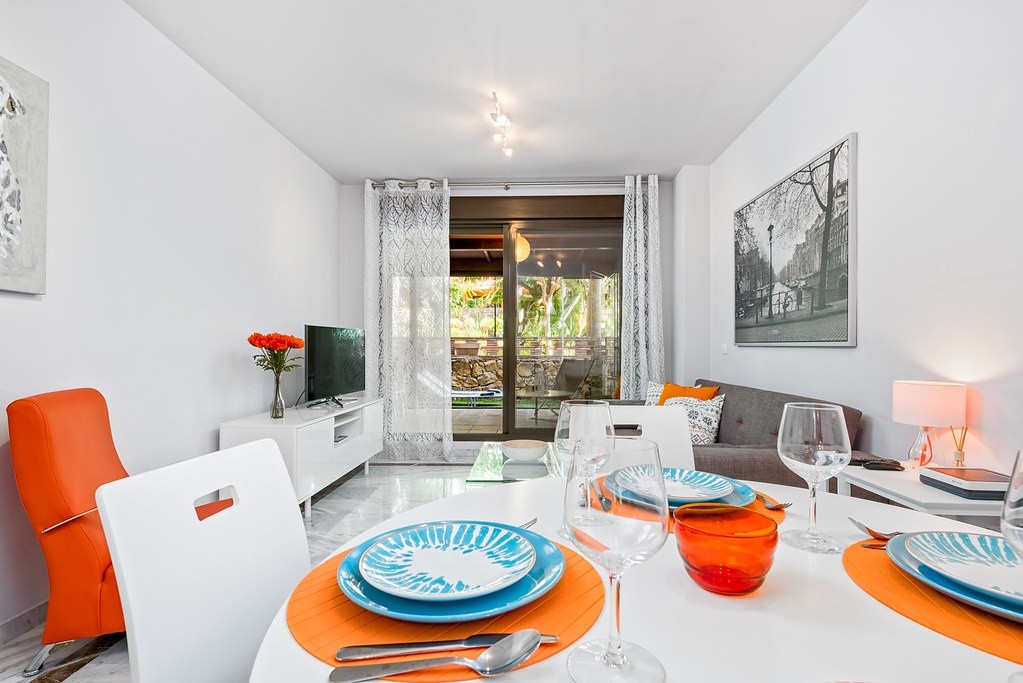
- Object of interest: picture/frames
[732,130,858,347]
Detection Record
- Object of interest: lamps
[515,232,530,262]
[891,379,967,466]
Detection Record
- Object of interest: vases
[269,373,285,418]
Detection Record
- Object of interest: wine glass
[564,435,670,683]
[777,402,851,554]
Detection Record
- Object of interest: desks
[832,461,1006,517]
[515,388,574,425]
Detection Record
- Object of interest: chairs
[5,387,235,677]
[533,358,596,419]
[94,436,310,683]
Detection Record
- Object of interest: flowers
[247,331,305,416]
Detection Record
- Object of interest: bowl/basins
[500,459,548,479]
[500,440,548,462]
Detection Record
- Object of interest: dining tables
[249,470,1023,683]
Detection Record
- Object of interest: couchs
[596,377,890,504]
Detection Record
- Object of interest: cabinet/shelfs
[218,394,385,518]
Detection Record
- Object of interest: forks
[757,493,792,510]
[848,517,906,540]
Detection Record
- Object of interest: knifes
[335,631,559,661]
[860,542,887,551]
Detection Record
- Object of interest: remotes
[849,458,901,467]
[863,461,905,472]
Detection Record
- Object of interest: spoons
[327,628,541,683]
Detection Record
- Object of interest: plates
[886,532,1023,624]
[615,465,733,504]
[603,470,756,512]
[337,521,564,622]
[906,532,1023,608]
[358,523,536,600]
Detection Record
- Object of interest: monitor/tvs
[304,324,366,408]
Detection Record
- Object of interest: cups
[553,399,615,474]
[1000,442,1023,586]
[673,503,778,596]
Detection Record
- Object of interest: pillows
[663,393,726,445]
[636,380,703,430]
[658,381,721,405]
